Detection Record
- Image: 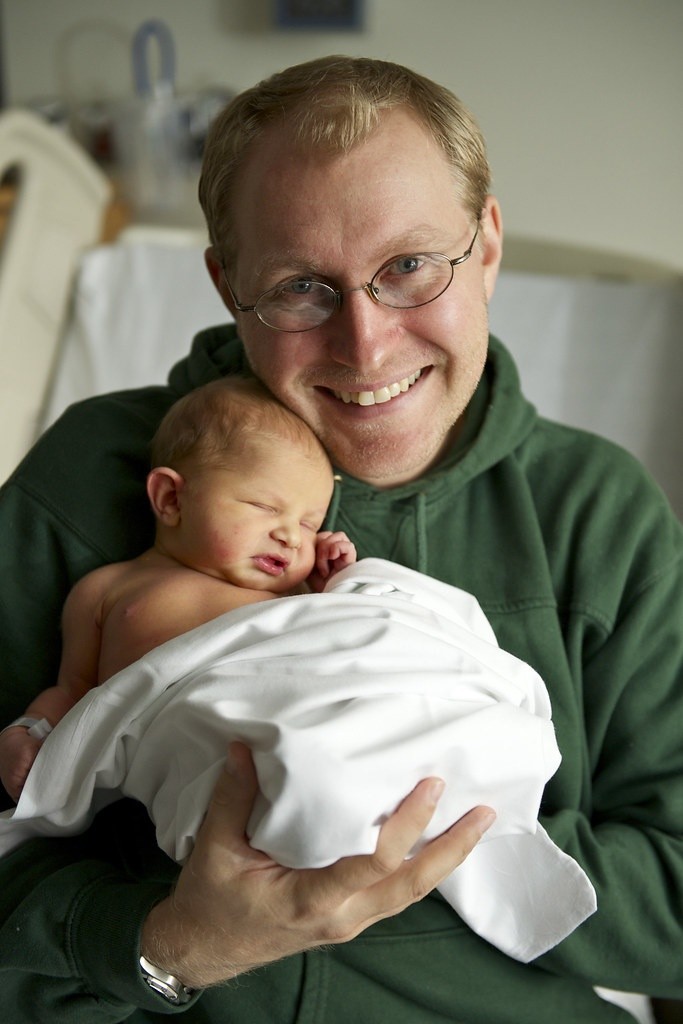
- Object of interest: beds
[0,108,683,524]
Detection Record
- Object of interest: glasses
[220,217,480,333]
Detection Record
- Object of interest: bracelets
[2,716,52,742]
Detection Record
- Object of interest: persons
[0,54,682,1024]
[0,375,563,869]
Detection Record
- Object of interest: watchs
[139,954,191,1005]
